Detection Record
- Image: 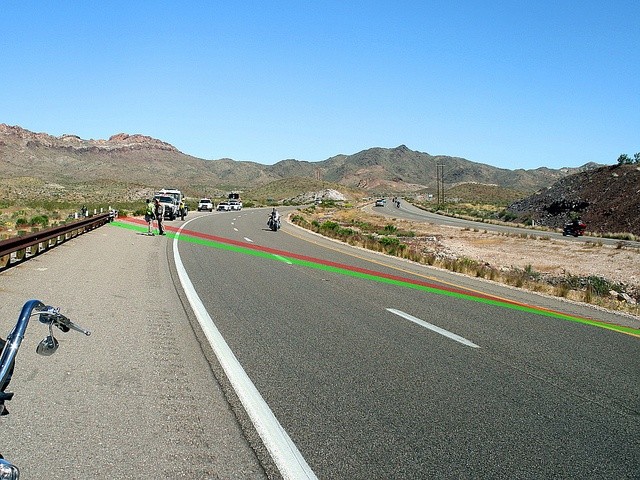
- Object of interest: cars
[216,202,231,211]
[376,200,384,207]
[228,199,242,211]
[382,199,386,203]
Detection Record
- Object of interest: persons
[82,204,88,216]
[571,215,582,227]
[153,199,166,234]
[180,198,185,221]
[145,199,156,236]
[268,206,280,225]
[397,200,401,207]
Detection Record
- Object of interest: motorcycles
[266,212,283,231]
[562,220,586,237]
[1,301,90,479]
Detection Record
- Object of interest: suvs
[197,198,213,212]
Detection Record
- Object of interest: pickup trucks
[152,193,177,221]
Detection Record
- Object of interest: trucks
[146,188,188,216]
